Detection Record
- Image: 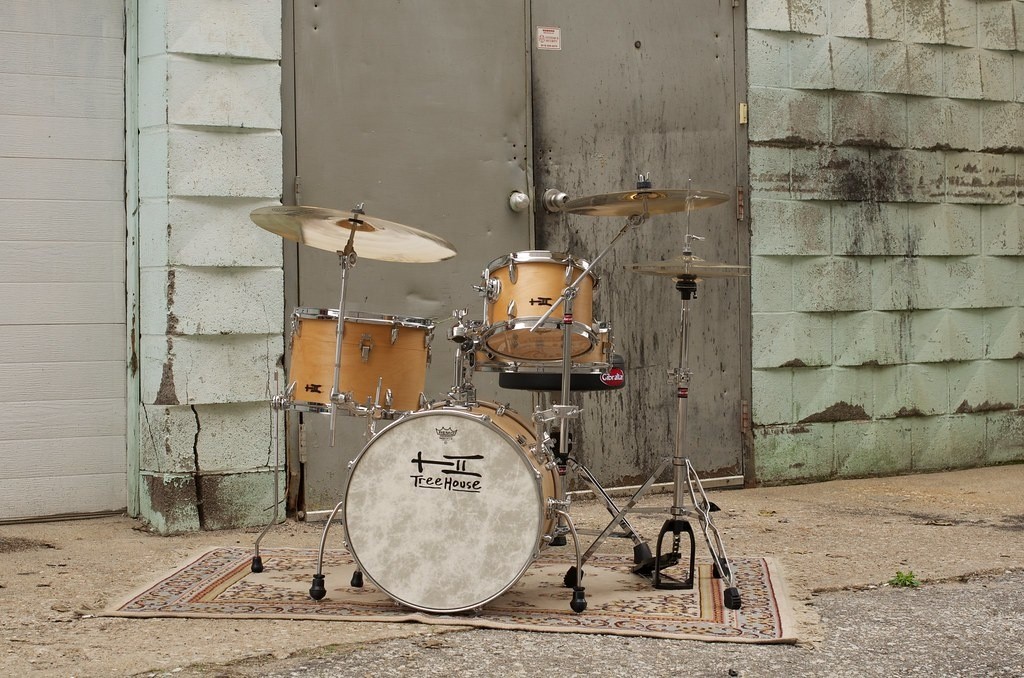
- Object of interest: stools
[498,353,652,567]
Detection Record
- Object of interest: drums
[469,317,615,375]
[271,305,434,422]
[472,248,603,366]
[342,395,565,612]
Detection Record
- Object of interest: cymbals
[249,204,457,264]
[624,253,752,279]
[558,189,732,217]
